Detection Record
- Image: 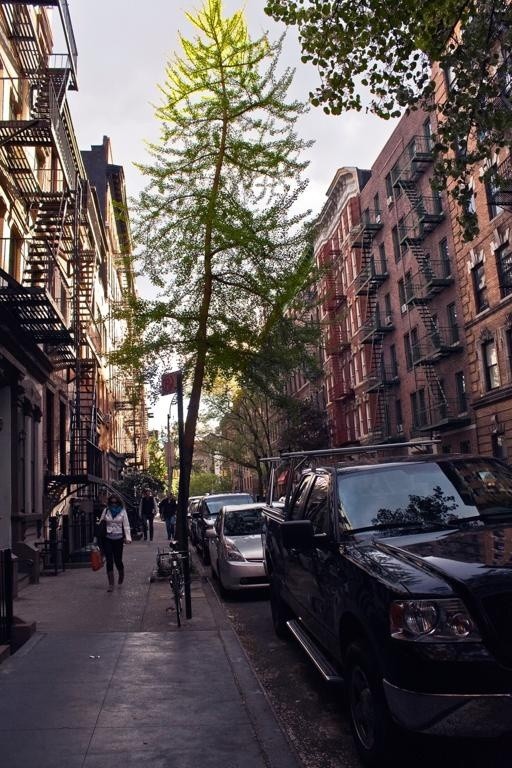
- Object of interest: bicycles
[155,540,194,630]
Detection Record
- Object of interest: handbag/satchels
[97,520,108,547]
[91,550,104,571]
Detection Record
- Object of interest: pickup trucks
[257,437,512,755]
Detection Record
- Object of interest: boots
[118,566,124,584]
[107,571,114,592]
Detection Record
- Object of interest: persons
[141,488,148,532]
[139,490,158,541]
[93,494,133,593]
[162,492,177,540]
[159,492,172,521]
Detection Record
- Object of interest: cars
[186,490,286,602]
